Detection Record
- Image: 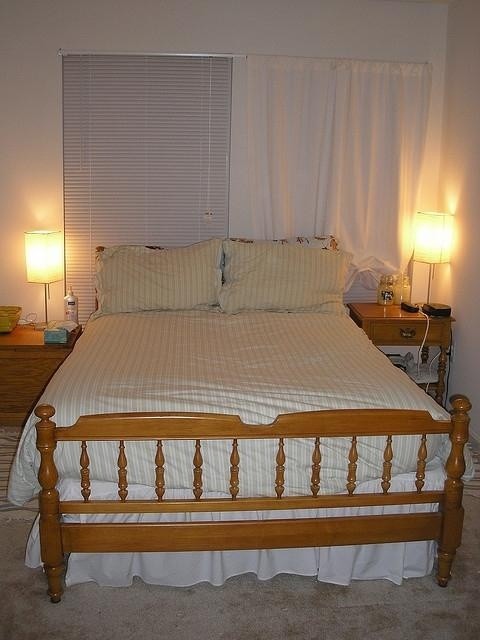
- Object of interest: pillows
[89,237,221,317]
[232,235,338,251]
[219,238,352,320]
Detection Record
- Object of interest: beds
[7,238,471,604]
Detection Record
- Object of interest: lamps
[26,231,65,330]
[413,211,458,307]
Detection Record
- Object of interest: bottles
[377,275,395,305]
[394,274,410,304]
[63,286,78,325]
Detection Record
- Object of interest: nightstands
[345,302,455,407]
[0,323,83,428]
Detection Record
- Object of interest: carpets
[0,424,39,525]
[461,442,480,499]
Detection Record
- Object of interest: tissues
[44,321,79,343]
